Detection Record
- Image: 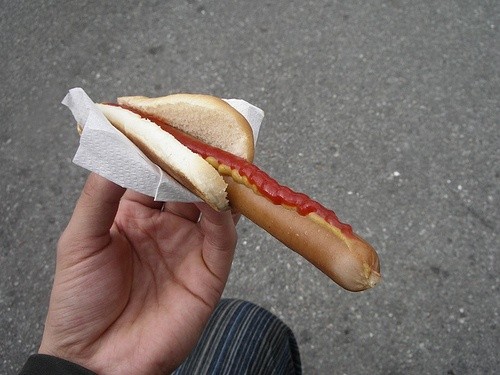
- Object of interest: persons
[19,172,301,375]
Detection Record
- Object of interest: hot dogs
[75,91,381,293]
